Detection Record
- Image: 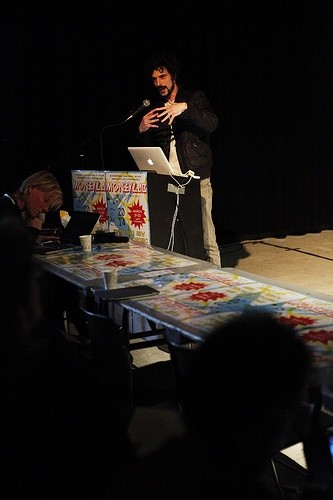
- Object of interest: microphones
[127,100,150,120]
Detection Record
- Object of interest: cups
[79,235,92,253]
[40,213,45,224]
[103,270,118,289]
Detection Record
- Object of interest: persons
[137,308,332,499]
[55,315,136,439]
[3,269,332,500]
[0,169,64,323]
[125,56,223,269]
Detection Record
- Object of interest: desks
[32,242,333,413]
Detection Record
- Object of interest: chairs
[74,307,136,373]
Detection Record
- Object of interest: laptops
[127,147,200,179]
[34,211,101,255]
